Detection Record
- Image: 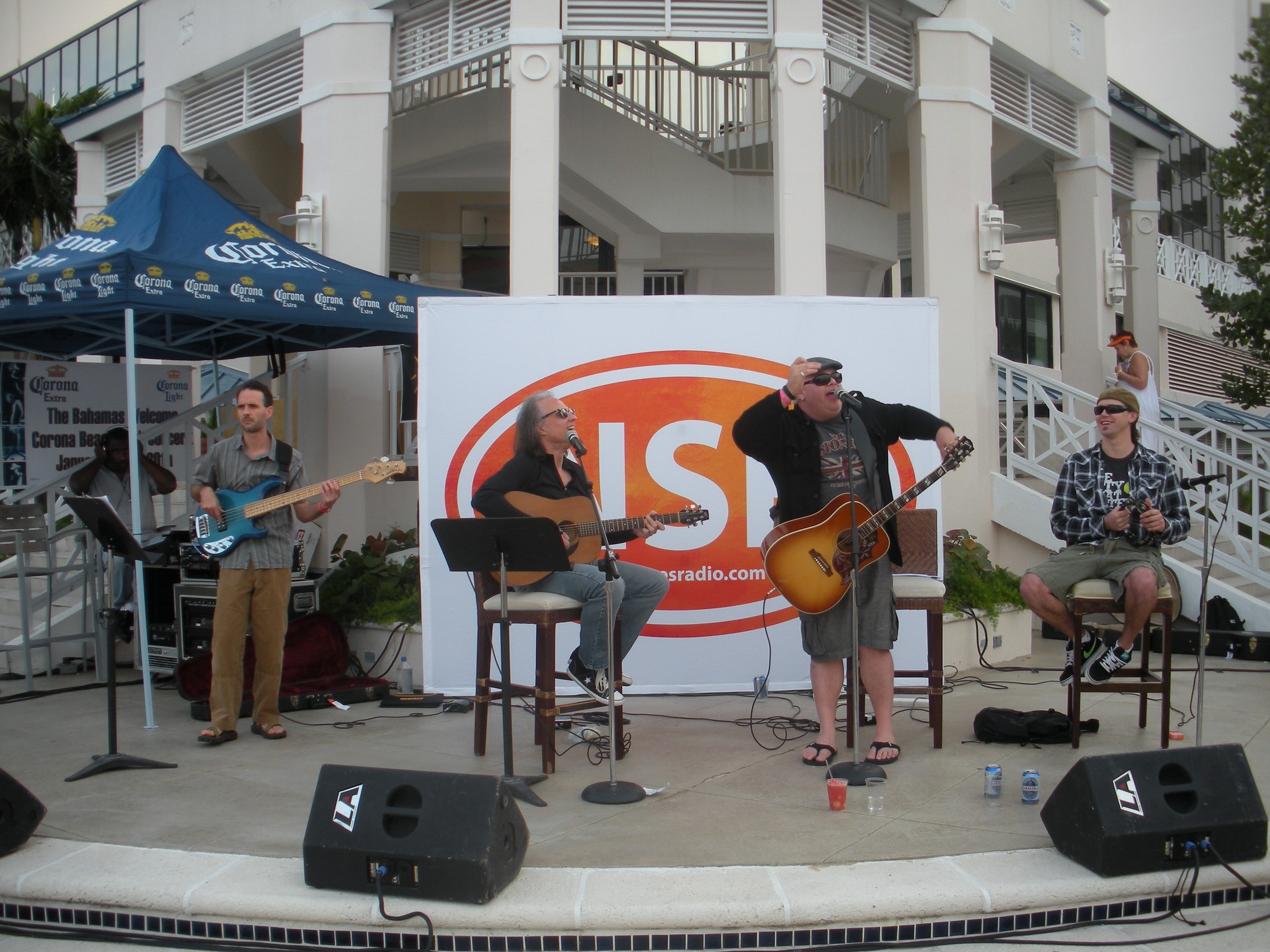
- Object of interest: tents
[0,145,500,745]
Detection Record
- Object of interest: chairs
[468,567,624,776]
[1063,540,1174,749]
[845,507,954,751]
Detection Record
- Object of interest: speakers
[1040,744,1268,877]
[0,769,46,859]
[302,762,530,905]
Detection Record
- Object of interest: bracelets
[782,385,797,400]
[778,389,791,406]
[318,501,332,514]
[1116,369,1123,376]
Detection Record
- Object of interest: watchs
[1018,387,1192,687]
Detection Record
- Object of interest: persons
[731,354,959,767]
[68,425,180,623]
[470,388,670,706]
[188,382,345,749]
[1103,329,1162,451]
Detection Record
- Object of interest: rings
[800,371,806,377]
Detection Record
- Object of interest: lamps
[976,202,1021,270]
[1104,247,1139,305]
[277,192,324,258]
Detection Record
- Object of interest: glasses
[803,373,843,387]
[539,408,576,419]
[1094,405,1133,415]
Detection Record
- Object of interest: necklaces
[110,467,131,502]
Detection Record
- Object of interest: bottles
[397,656,413,694]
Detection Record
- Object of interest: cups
[826,778,848,810]
[865,777,886,810]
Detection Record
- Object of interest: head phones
[100,425,129,454]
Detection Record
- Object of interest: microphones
[1180,474,1226,490]
[567,430,588,456]
[833,387,862,411]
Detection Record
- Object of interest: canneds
[1021,770,1040,804]
[983,765,1002,800]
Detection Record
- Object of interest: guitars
[194,456,408,558]
[759,435,975,614]
[484,490,709,586]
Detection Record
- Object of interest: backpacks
[973,706,1080,744]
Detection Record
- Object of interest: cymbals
[1116,496,1158,549]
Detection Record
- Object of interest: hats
[1097,387,1140,413]
[1107,335,1132,347]
[806,357,843,372]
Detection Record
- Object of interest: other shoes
[120,598,135,614]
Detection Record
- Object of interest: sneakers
[1059,628,1106,686]
[1084,639,1134,685]
[566,655,625,706]
[568,645,633,687]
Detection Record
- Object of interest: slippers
[198,726,238,744]
[864,741,901,765]
[251,721,287,740]
[802,741,838,766]
